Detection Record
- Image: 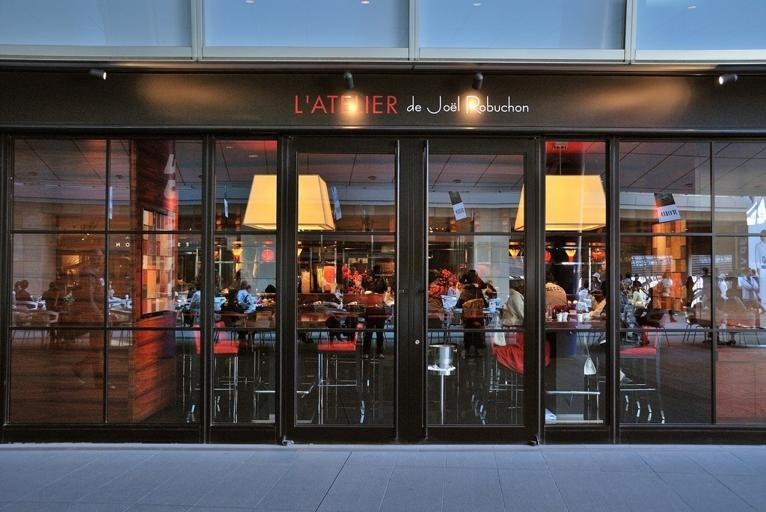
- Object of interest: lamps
[242,172,337,234]
[89,68,108,80]
[471,71,484,92]
[514,142,607,232]
[718,74,739,85]
[341,71,356,91]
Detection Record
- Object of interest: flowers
[342,263,363,290]
[430,268,462,300]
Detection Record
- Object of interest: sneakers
[708,333,737,344]
[362,354,386,359]
[670,318,678,323]
[72,367,117,391]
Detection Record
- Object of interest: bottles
[719,321,729,340]
[545,302,590,324]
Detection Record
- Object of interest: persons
[324,265,393,358]
[756,231,766,313]
[13,279,62,343]
[173,277,259,344]
[430,269,524,373]
[545,270,766,383]
[72,251,115,390]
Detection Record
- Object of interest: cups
[440,296,500,327]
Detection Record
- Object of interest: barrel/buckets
[429,344,454,370]
